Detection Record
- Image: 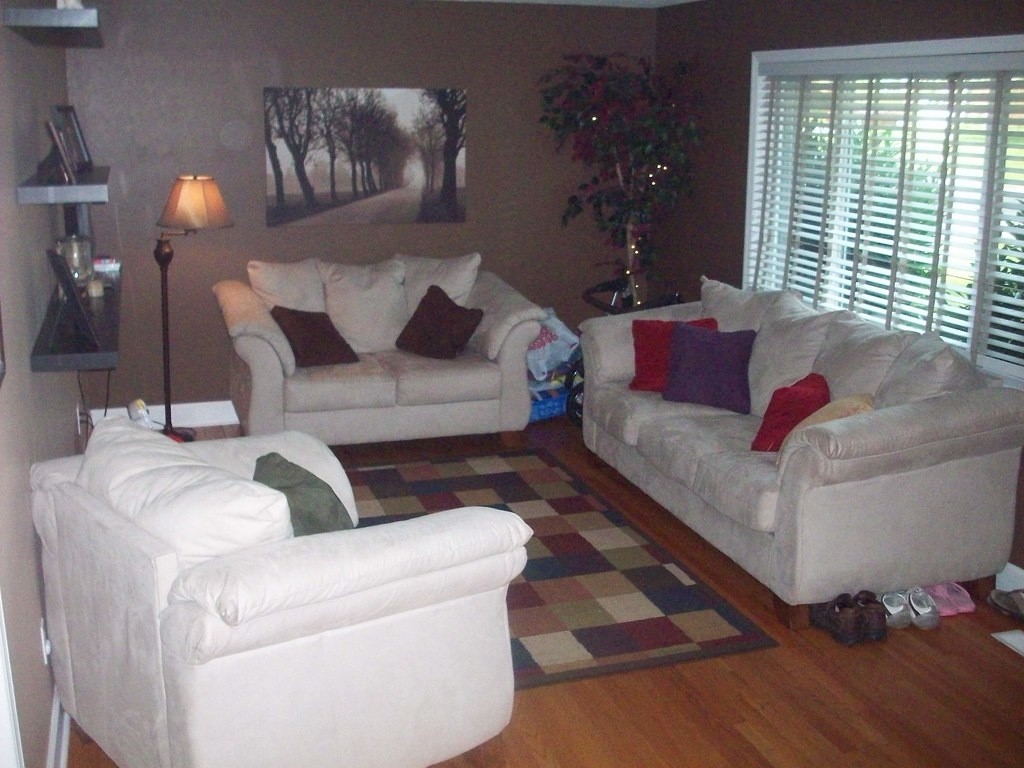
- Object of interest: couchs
[29,430,535,768]
[577,303,1023,631]
[213,270,548,447]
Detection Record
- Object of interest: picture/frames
[47,105,92,184]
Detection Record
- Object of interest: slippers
[926,580,975,616]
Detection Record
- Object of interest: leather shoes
[810,590,887,643]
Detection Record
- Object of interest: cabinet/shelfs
[2,8,122,371]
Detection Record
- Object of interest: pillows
[247,257,326,312]
[272,305,360,366]
[253,452,354,539]
[750,372,830,452]
[661,320,755,415]
[395,285,483,360]
[392,253,481,315]
[809,310,921,404]
[872,330,987,410]
[628,319,718,392]
[776,395,872,465]
[700,275,802,333]
[747,290,847,417]
[315,260,407,353]
[74,417,294,570]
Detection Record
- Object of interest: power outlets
[77,404,87,434]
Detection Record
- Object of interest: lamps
[154,175,235,442]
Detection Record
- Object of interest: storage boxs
[529,387,570,425]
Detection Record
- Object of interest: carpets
[990,630,1024,657]
[341,447,781,689]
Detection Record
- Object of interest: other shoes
[876,585,943,628]
[987,586,1024,617]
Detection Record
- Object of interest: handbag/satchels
[526,306,579,381]
